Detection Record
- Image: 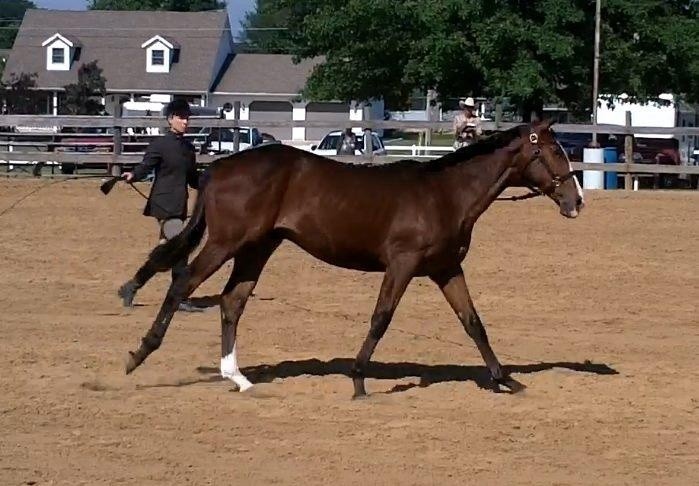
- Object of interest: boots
[117,277,140,308]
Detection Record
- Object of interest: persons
[119,100,206,310]
[619,141,643,190]
[359,128,387,155]
[452,97,483,153]
[656,141,679,189]
[336,123,357,165]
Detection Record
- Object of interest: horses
[126,115,585,399]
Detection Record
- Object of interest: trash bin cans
[584,146,617,189]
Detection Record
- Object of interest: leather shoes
[174,296,201,314]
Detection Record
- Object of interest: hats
[165,99,191,117]
[459,96,482,112]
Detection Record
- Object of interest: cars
[51,116,271,187]
[488,123,698,191]
[308,123,387,156]
